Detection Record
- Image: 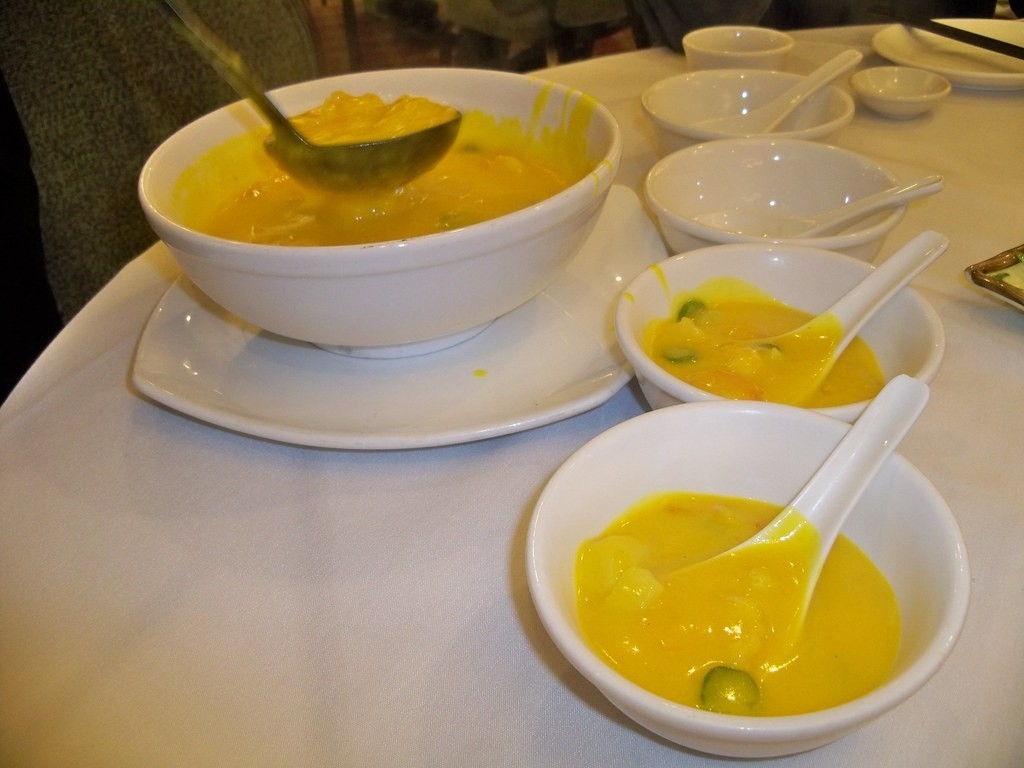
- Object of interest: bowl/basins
[618,241,948,425]
[525,400,968,759]
[682,27,796,77]
[645,136,907,265]
[137,69,626,361]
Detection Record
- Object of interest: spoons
[692,174,946,240]
[750,230,952,399]
[673,374,931,642]
[692,49,864,134]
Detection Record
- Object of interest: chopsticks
[869,5,1024,62]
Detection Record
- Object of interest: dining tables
[0,20,1024,767]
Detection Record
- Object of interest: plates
[132,183,671,452]
[645,67,855,141]
[963,243,1024,311]
[874,17,1024,93]
[850,65,956,117]
